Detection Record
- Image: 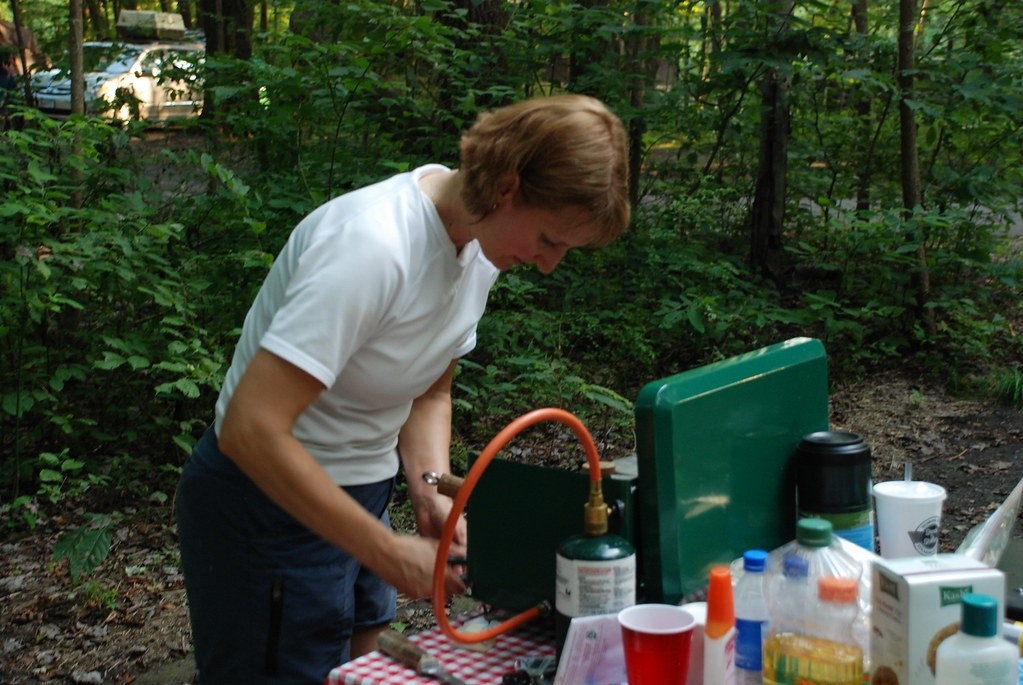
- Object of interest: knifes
[378,628,466,685]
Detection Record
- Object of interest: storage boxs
[869,550,1004,685]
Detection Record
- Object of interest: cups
[618,603,697,685]
[873,481,948,559]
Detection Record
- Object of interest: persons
[175,94,632,685]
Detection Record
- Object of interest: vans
[24,41,205,135]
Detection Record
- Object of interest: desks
[323,601,556,685]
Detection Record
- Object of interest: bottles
[935,592,1019,685]
[763,517,874,685]
[730,549,772,685]
[703,567,738,685]
